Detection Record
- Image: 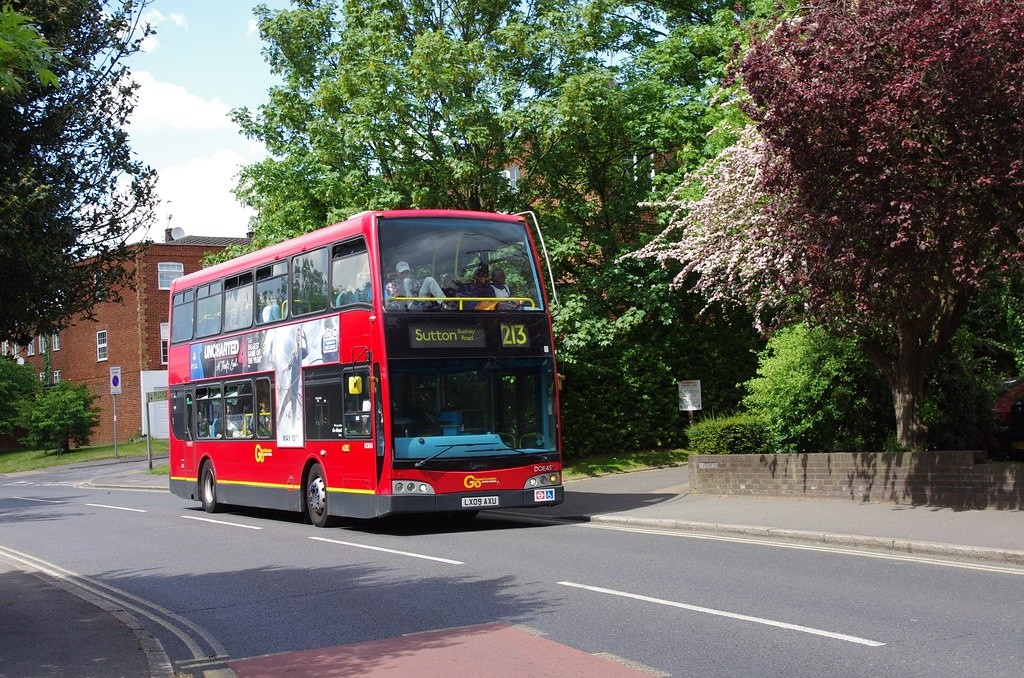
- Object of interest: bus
[167,208,568,530]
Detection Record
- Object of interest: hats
[395,261,411,273]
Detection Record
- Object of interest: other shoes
[440,303,457,311]
[406,300,420,311]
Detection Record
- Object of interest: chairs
[210,419,219,437]
[191,282,487,339]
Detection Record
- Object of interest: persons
[360,383,396,436]
[211,399,271,438]
[257,259,524,321]
[277,318,339,428]
